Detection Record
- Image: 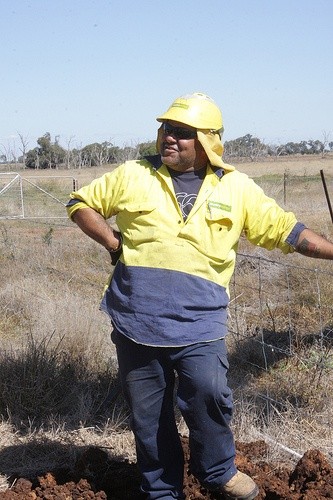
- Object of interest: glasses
[161,122,197,139]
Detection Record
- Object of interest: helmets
[156,93,223,133]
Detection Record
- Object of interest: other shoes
[219,468,259,500]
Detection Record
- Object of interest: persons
[64,93,333,500]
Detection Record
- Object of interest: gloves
[110,231,123,267]
